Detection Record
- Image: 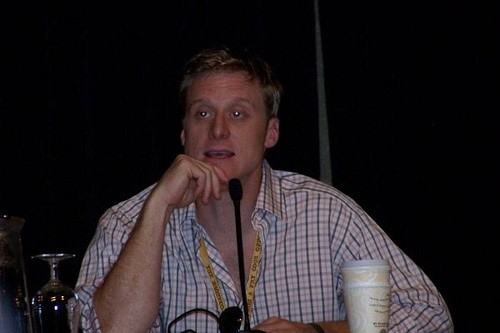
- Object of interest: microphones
[228,177,266,333]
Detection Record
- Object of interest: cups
[341,260,391,333]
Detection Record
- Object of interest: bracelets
[307,322,325,333]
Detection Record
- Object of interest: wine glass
[32,253,80,332]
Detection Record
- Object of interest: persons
[66,46,454,333]
